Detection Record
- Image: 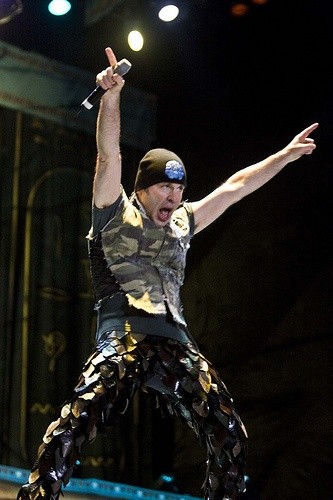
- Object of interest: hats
[134,148,187,193]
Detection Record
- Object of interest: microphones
[81,59,132,112]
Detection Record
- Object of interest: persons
[17,47,318,499]
